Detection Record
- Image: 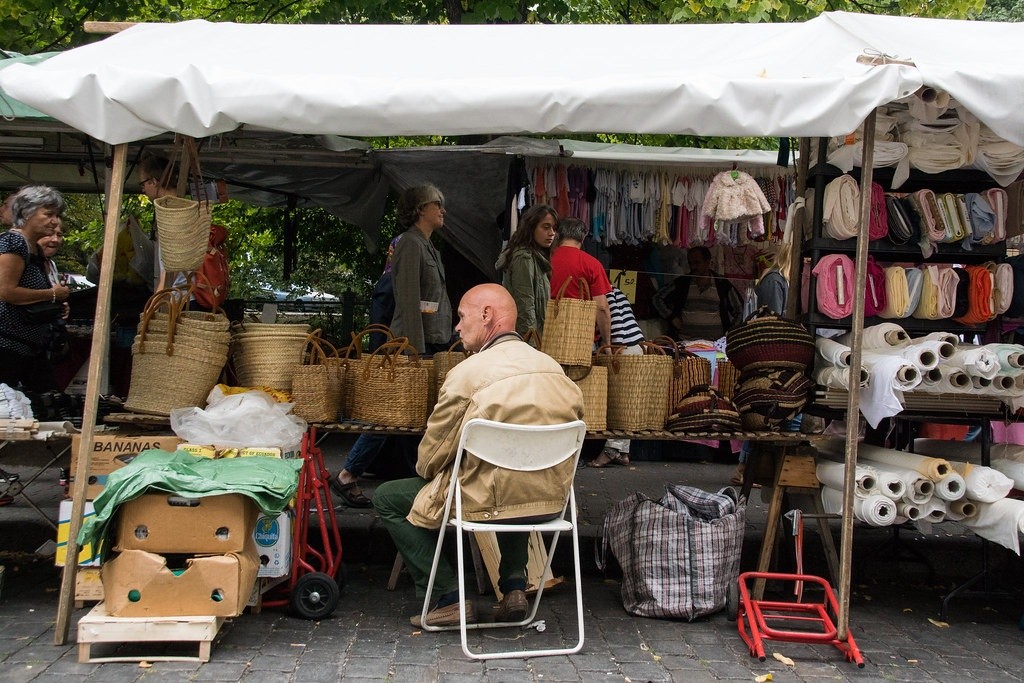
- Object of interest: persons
[373,283,583,626]
[730,201,806,489]
[0,186,89,504]
[135,149,216,328]
[328,184,649,511]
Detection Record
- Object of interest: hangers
[523,154,798,181]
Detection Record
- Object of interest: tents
[0,11,1024,236]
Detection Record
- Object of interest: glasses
[422,200,447,212]
[139,177,158,189]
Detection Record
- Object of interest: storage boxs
[69,434,185,500]
[115,488,263,557]
[56,500,104,569]
[253,503,298,579]
[99,546,262,616]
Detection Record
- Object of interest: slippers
[589,457,629,465]
[593,448,621,468]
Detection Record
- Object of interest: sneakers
[0,468,19,482]
[59,470,70,486]
[0,490,15,505]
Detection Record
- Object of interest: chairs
[422,420,588,660]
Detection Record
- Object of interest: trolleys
[62,420,344,622]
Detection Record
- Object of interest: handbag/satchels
[157,137,211,271]
[594,482,747,621]
[125,270,741,431]
[85,215,157,285]
[19,301,66,326]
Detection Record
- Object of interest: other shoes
[577,460,586,469]
[494,590,528,625]
[410,600,474,626]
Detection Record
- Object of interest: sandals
[330,471,373,509]
[730,468,762,487]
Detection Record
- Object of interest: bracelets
[51,288,57,303]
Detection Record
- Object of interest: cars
[233,248,341,312]
